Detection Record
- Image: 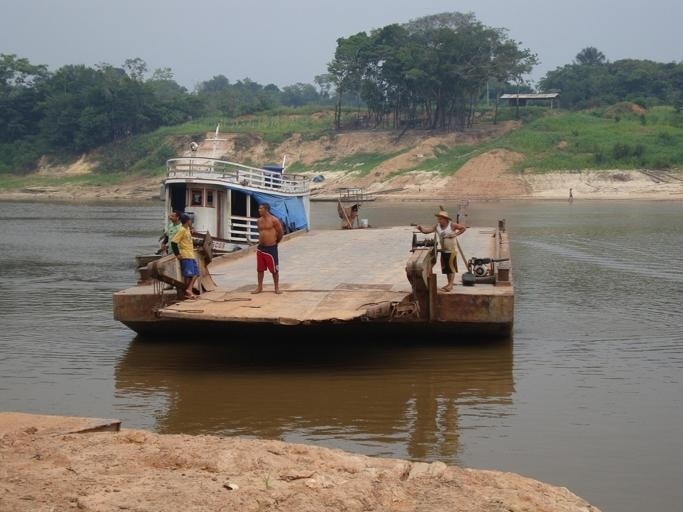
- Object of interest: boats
[306,186,378,202]
[131,124,317,276]
[107,216,523,351]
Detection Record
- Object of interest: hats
[180,212,195,222]
[434,210,452,221]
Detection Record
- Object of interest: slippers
[440,285,453,292]
[184,294,200,300]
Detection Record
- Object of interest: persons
[415,211,467,292]
[170,213,200,300]
[567,188,573,202]
[250,203,284,294]
[160,209,183,290]
